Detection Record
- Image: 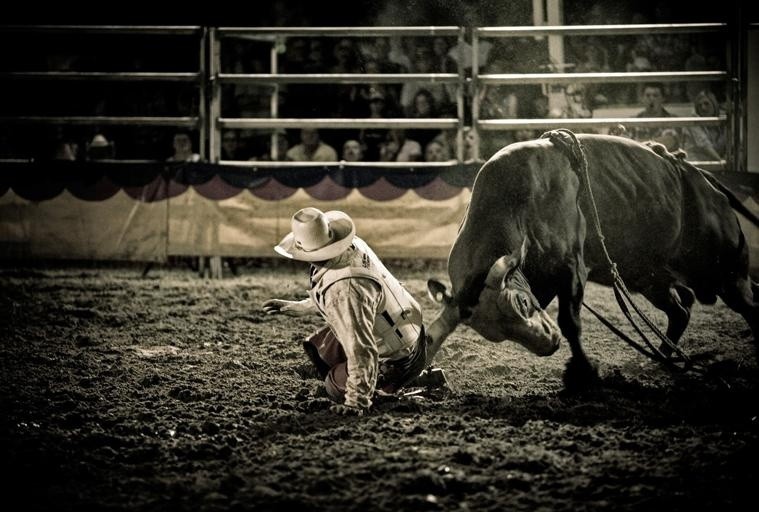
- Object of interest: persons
[259,204,445,417]
[0,0,747,168]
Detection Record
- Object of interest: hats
[274,207,356,262]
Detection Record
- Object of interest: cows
[379,133,759,394]
